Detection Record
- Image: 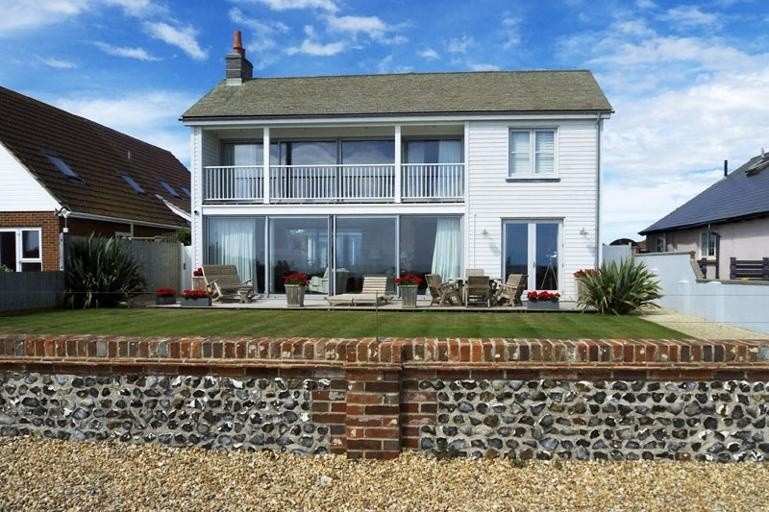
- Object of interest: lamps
[63,218,68,232]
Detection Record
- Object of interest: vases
[400,285,419,310]
[578,277,587,297]
[191,276,203,289]
[284,283,306,306]
[526,300,559,310]
[154,296,212,308]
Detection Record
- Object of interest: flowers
[194,267,203,277]
[394,273,424,286]
[574,269,598,278]
[155,288,214,299]
[284,272,310,286]
[527,291,560,303]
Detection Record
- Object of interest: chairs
[325,277,388,307]
[425,268,528,307]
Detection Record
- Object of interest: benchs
[198,264,257,304]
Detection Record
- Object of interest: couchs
[308,267,350,294]
